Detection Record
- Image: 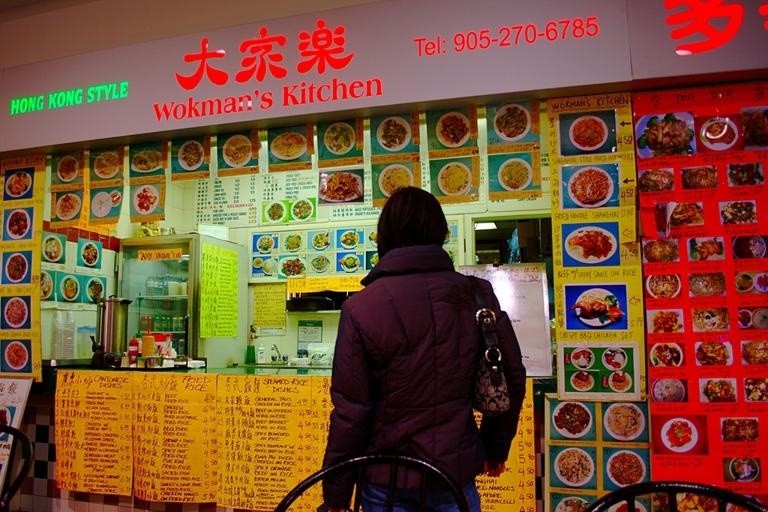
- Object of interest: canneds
[601,374,608,389]
[141,315,185,332]
[178,339,186,356]
[564,353,571,365]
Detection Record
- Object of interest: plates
[606,449,647,487]
[178,140,205,172]
[570,372,595,392]
[568,115,609,150]
[603,402,646,440]
[252,230,379,273]
[601,347,627,371]
[575,288,619,328]
[567,166,614,209]
[551,402,593,440]
[0,406,12,437]
[290,199,315,221]
[437,162,472,195]
[93,152,120,180]
[91,191,113,217]
[324,122,356,156]
[497,158,532,191]
[608,371,633,393]
[553,447,595,486]
[271,132,307,160]
[564,226,618,264]
[265,201,287,222]
[222,134,253,168]
[130,150,163,173]
[133,184,159,215]
[56,156,79,181]
[494,104,532,142]
[2,171,36,376]
[435,112,471,148]
[56,193,82,221]
[378,163,414,198]
[376,116,412,151]
[37,238,107,305]
[319,172,363,202]
[571,348,595,371]
[637,107,766,482]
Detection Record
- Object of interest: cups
[297,342,309,358]
[51,311,75,358]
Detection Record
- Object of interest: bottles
[141,328,156,357]
[257,343,265,363]
[128,335,138,365]
[136,358,145,368]
[143,271,188,297]
[121,351,129,368]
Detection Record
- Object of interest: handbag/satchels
[475,345,511,416]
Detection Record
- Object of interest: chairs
[0,421,35,512]
[272,451,469,512]
[582,479,768,511]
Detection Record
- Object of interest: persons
[321,186,525,512]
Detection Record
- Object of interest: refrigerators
[116,230,252,365]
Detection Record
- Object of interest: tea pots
[94,293,133,363]
